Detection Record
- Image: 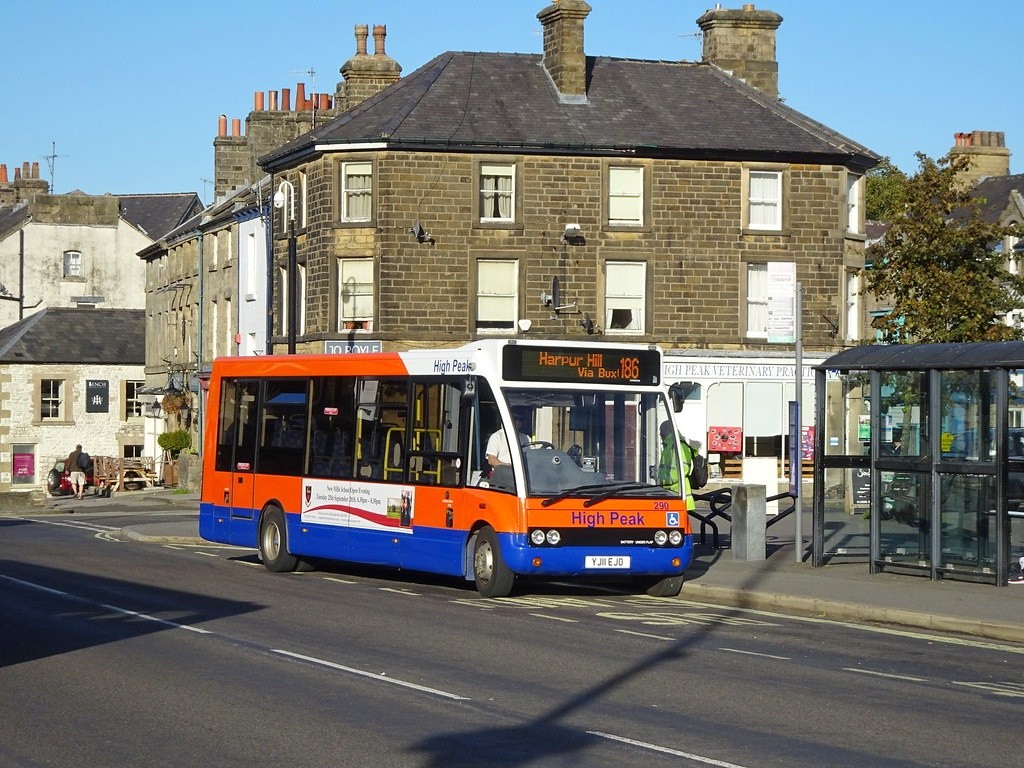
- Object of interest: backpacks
[672,440,708,490]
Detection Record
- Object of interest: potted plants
[160,394,189,414]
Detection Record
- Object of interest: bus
[198,338,696,598]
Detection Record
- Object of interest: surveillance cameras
[273,191,285,208]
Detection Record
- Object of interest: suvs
[46,456,111,498]
[950,425,1024,513]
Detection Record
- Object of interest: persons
[657,420,696,511]
[65,444,88,500]
[400,495,411,527]
[486,412,537,472]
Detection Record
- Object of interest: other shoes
[73,493,77,498]
[78,496,82,499]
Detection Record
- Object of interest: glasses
[512,415,523,422]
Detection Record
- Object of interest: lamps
[559,224,581,245]
[408,221,431,241]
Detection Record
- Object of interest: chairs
[224,413,432,486]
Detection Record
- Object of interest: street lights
[274,180,299,354]
